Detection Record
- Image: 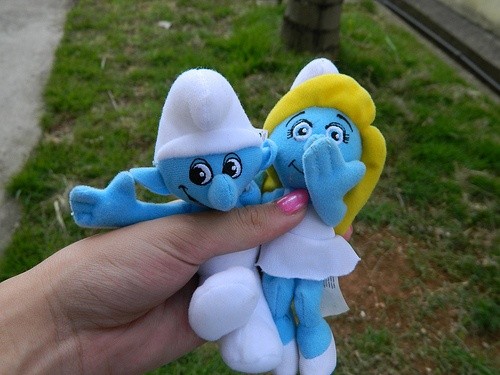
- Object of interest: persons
[0,189,324,375]
